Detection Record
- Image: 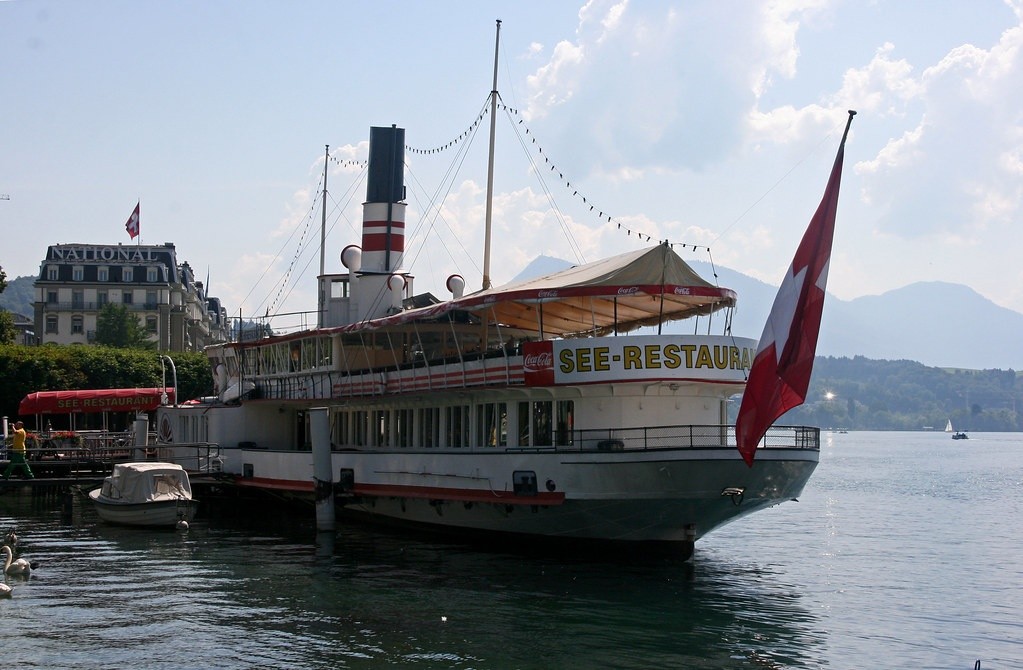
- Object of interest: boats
[87,460,201,532]
[952,433,969,439]
[155,14,857,562]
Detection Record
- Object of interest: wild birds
[0,526,31,595]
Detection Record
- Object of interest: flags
[734,149,844,469]
[125,202,140,240]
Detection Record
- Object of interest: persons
[128,421,133,431]
[46,419,52,430]
[518,336,531,355]
[0,421,34,479]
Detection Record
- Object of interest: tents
[18,387,176,453]
[338,242,738,370]
